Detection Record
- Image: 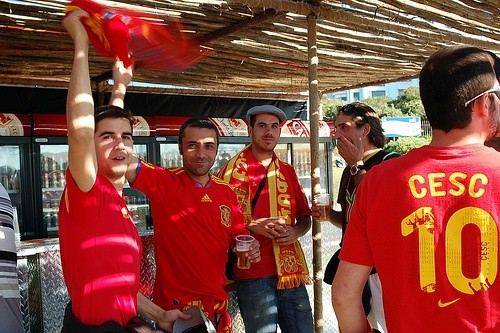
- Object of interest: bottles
[42,191,61,227]
[1,160,19,190]
[277,150,311,175]
[162,154,183,168]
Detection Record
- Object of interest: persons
[216,105,313,333]
[313,101,401,333]
[56,9,192,333]
[331,46,500,333]
[104,56,261,333]
[0,181,25,333]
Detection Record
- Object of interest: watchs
[350,163,365,176]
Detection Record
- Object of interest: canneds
[0,147,69,227]
[123,145,183,205]
[276,151,311,176]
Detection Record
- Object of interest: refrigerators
[0,113,335,243]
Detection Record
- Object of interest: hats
[246,105,286,123]
[173,306,216,333]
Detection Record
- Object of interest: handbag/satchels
[225,246,238,280]
[323,247,342,285]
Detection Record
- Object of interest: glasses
[464,86,500,107]
[336,104,368,122]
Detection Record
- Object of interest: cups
[270,216,288,244]
[313,193,331,222]
[234,234,255,269]
